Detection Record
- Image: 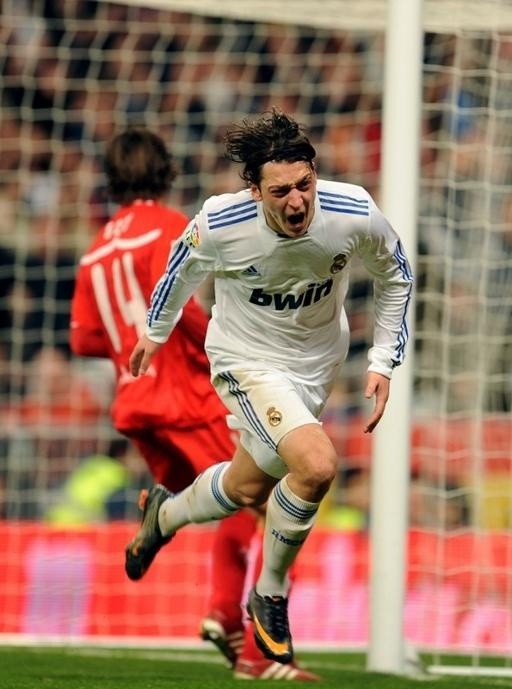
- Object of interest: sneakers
[233,655,318,681]
[198,608,246,667]
[247,586,293,663]
[125,484,175,580]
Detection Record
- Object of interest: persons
[73,122,323,682]
[0,0,512,537]
[116,108,414,666]
